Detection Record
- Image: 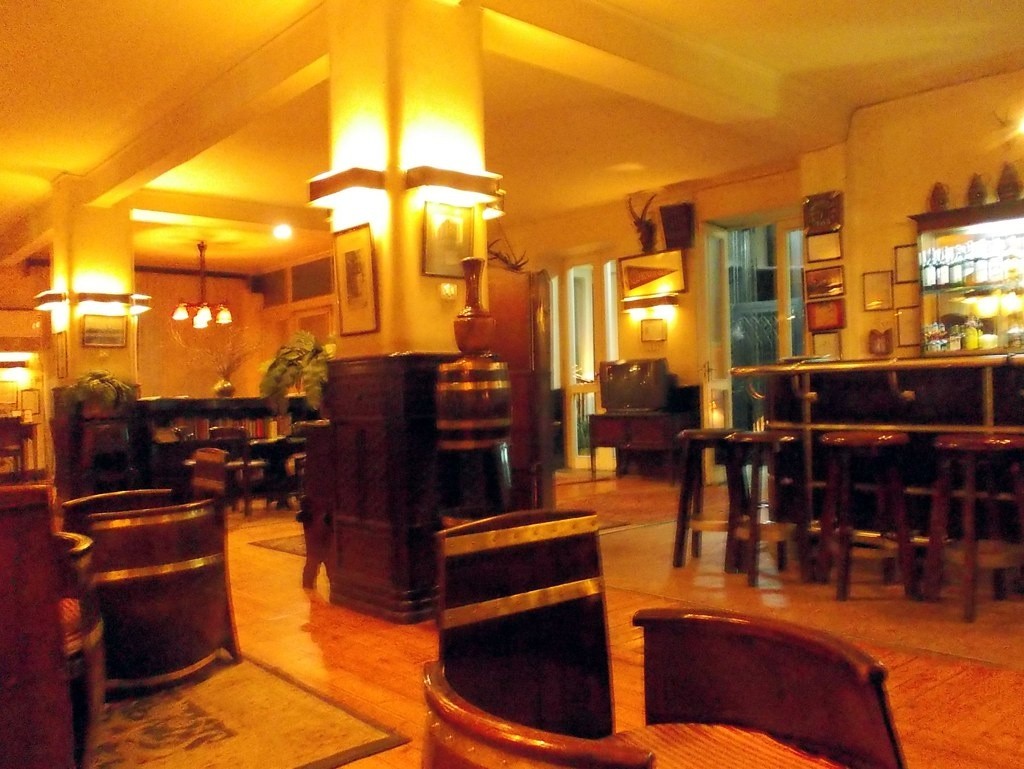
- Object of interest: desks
[727,354,1023,548]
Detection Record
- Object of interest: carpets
[82,654,414,769]
[247,477,729,557]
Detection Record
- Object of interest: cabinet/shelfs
[905,197,1024,356]
[302,421,337,590]
[487,266,540,507]
[589,411,690,489]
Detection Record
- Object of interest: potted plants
[57,371,142,423]
[258,329,337,423]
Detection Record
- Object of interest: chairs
[0,416,267,769]
[420,507,911,769]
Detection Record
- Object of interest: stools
[672,428,1023,626]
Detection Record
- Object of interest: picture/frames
[422,201,475,279]
[80,314,129,349]
[56,330,69,380]
[332,223,381,337]
[0,382,40,415]
[805,264,846,301]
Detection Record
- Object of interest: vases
[454,256,495,359]
[214,375,235,398]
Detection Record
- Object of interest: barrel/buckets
[433,361,513,450]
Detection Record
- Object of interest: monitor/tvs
[599,357,670,414]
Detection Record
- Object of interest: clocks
[803,191,844,236]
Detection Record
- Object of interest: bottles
[924,316,982,353]
[921,231,1023,295]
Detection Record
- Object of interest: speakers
[660,203,695,251]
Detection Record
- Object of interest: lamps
[404,163,507,223]
[173,240,233,329]
[309,165,387,207]
[32,289,152,315]
